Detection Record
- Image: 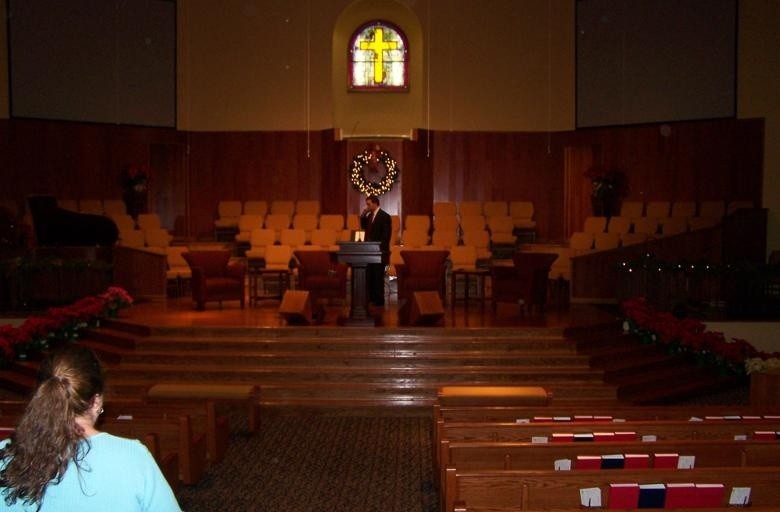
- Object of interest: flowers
[618,295,779,373]
[0,285,134,368]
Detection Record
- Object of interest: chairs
[488,251,559,320]
[0,197,191,300]
[547,199,755,309]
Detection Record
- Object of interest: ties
[367,212,374,228]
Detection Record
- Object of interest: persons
[359,195,393,307]
[0,349,183,512]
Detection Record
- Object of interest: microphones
[357,215,361,242]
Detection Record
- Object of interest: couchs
[180,249,248,311]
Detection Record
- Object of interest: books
[516,412,780,511]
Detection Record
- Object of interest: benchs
[1,382,260,512]
